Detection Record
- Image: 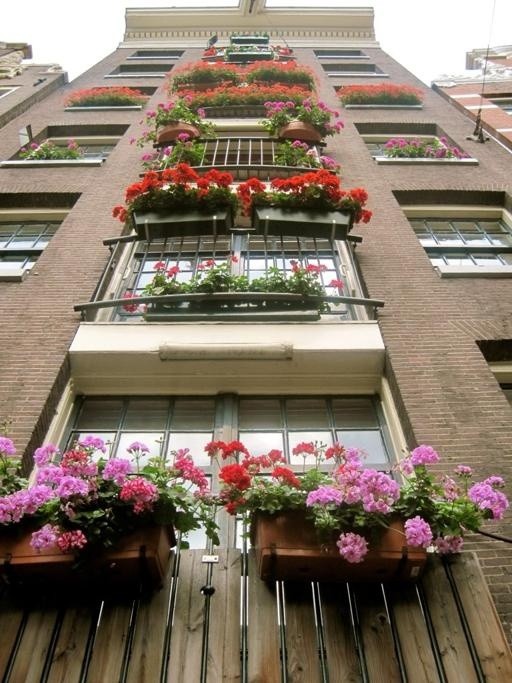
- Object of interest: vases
[249,509,427,582]
[131,206,234,239]
[142,309,320,322]
[251,202,354,240]
[0,506,177,590]
[276,121,320,142]
[155,124,200,144]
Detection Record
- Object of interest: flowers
[165,45,320,108]
[382,133,471,159]
[64,86,149,107]
[112,163,373,223]
[18,139,84,160]
[204,440,511,563]
[258,101,345,134]
[0,436,211,564]
[283,143,340,171]
[129,95,218,144]
[335,82,426,105]
[140,134,200,168]
[121,255,344,312]
